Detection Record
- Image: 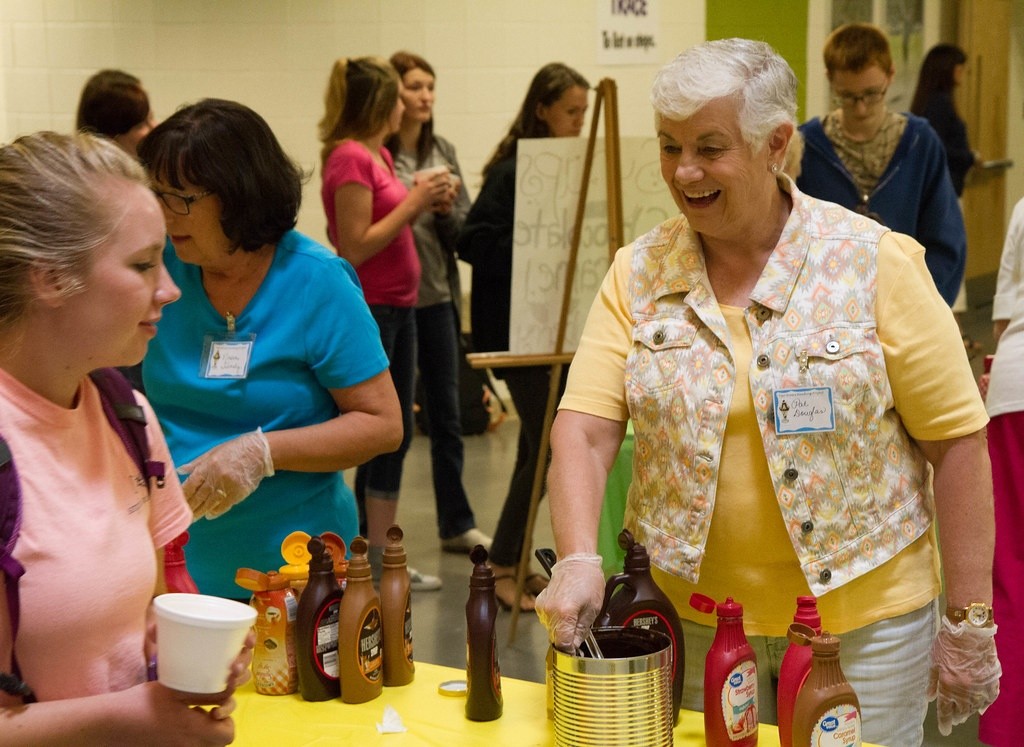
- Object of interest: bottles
[592,527,686,728]
[465,544,503,723]
[161,530,200,595]
[318,531,350,593]
[777,595,826,747]
[545,643,554,721]
[234,567,299,696]
[296,536,344,702]
[689,592,759,747]
[378,525,416,686]
[786,623,862,747]
[278,530,313,599]
[338,536,383,705]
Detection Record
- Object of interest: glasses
[150,186,215,215]
[830,65,889,109]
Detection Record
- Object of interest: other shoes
[441,529,493,553]
[370,567,442,590]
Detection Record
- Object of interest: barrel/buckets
[549,625,675,747]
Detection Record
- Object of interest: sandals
[492,575,536,611]
[517,572,548,594]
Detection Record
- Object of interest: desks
[179,657,886,747]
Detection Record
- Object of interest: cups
[413,165,451,212]
[152,592,258,695]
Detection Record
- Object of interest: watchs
[946,603,994,628]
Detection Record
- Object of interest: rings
[216,489,227,498]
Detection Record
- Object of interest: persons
[795,21,967,310]
[384,50,492,554]
[78,70,156,161]
[317,55,441,596]
[531,37,1002,747]
[137,96,404,602]
[975,195,1024,747]
[912,44,981,311]
[0,131,257,747]
[459,62,591,610]
